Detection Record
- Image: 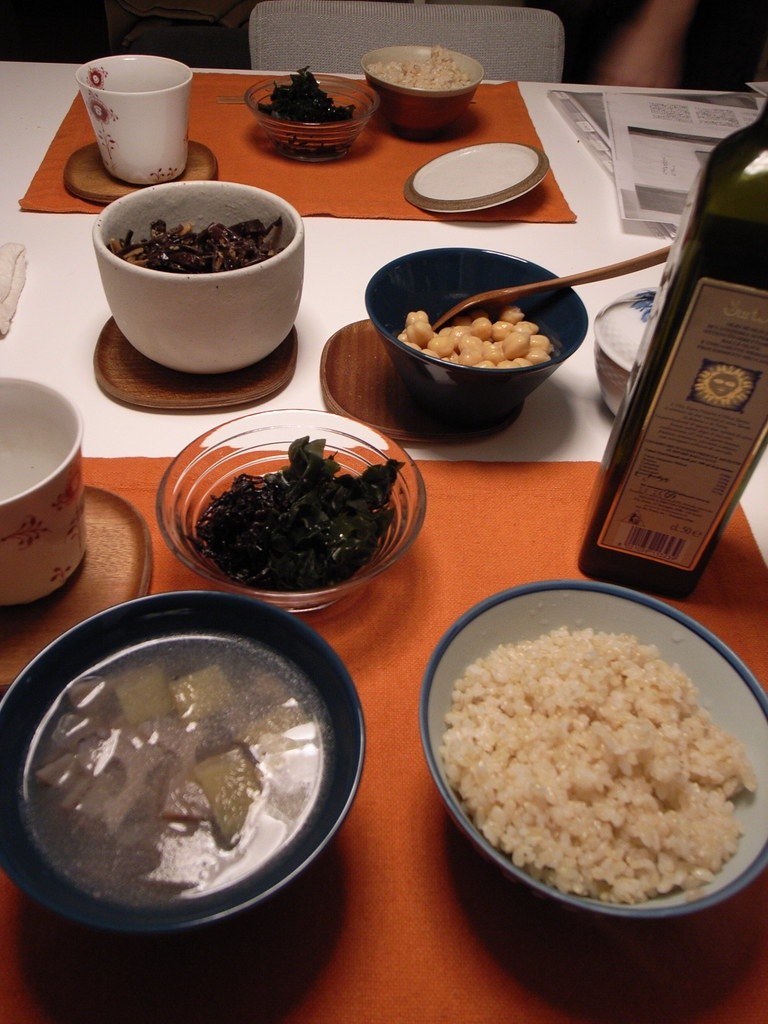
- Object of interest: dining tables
[0,61,768,1024]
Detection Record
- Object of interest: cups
[75,54,194,184]
[0,374,86,603]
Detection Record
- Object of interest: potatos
[112,662,307,845]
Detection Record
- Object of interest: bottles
[578,99,768,602]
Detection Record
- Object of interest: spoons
[433,242,674,334]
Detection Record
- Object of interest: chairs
[247,0,565,90]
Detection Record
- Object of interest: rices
[366,45,471,91]
[439,627,755,905]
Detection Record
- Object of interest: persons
[526,0,768,87]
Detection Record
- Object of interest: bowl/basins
[361,45,485,131]
[0,590,365,936]
[93,180,305,373]
[157,408,427,613]
[244,74,381,161]
[366,247,589,420]
[418,579,768,918]
[593,285,667,418]
